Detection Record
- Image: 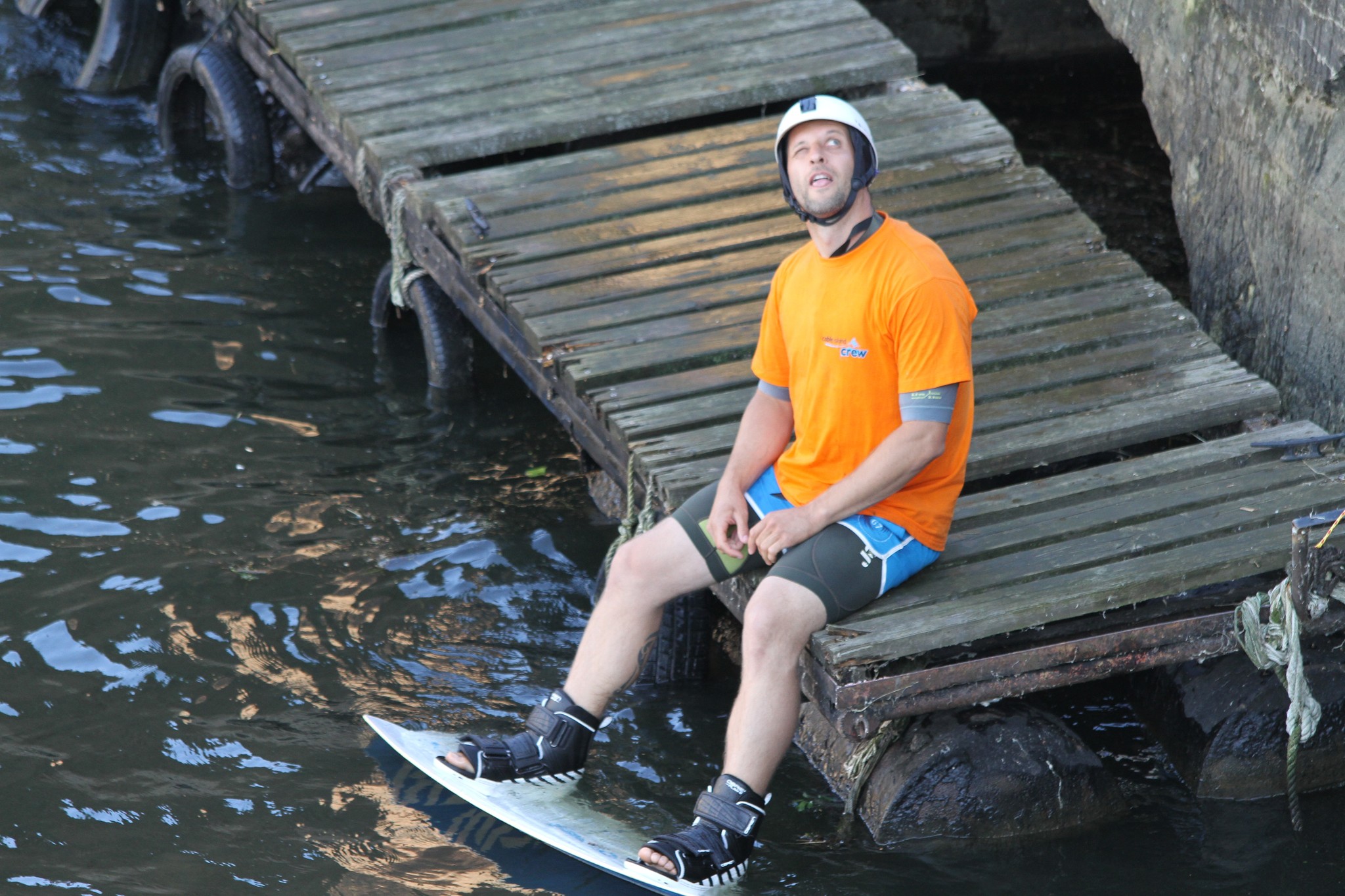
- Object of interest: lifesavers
[20,0,165,92]
[367,262,475,388]
[159,40,270,195]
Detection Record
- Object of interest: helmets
[774,95,879,189]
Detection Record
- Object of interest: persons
[436,94,978,888]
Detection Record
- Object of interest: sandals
[625,774,772,895]
[434,688,613,797]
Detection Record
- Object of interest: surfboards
[356,690,770,896]
